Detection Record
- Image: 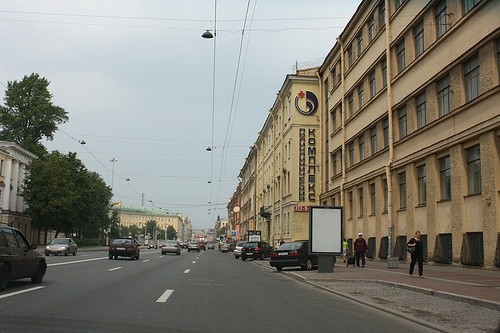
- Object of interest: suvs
[0,221,47,291]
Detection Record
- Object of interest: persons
[407,230,424,278]
[342,238,349,263]
[354,232,368,268]
[280,239,285,246]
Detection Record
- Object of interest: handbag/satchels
[406,246,416,254]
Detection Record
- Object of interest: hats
[358,233,362,236]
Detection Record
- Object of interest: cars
[241,240,274,261]
[45,238,78,256]
[162,240,181,255]
[269,239,337,271]
[147,241,157,249]
[219,244,234,252]
[188,241,215,253]
[108,236,141,260]
[234,241,248,259]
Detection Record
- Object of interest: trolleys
[346,254,357,268]
